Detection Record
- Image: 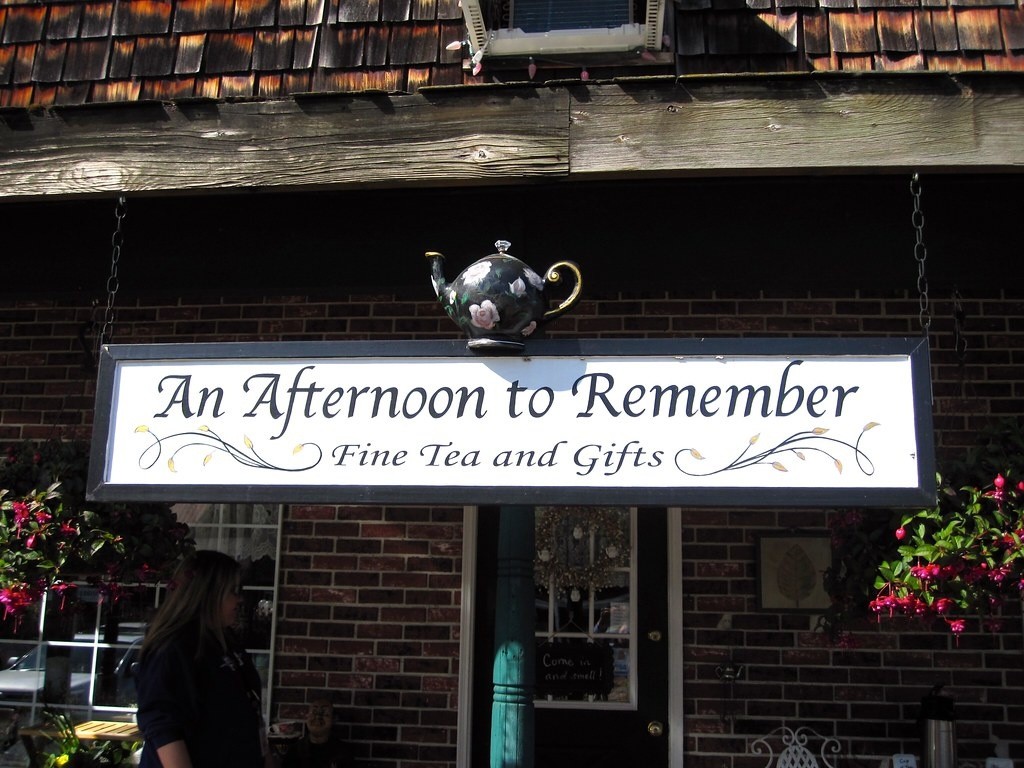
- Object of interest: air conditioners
[460,1,669,60]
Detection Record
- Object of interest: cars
[0,639,144,709]
[96,621,154,635]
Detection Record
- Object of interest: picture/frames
[752,528,836,614]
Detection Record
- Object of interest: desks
[19,719,145,768]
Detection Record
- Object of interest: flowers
[0,447,195,633]
[821,417,1024,654]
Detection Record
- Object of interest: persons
[135,551,263,767]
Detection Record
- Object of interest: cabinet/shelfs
[0,501,282,768]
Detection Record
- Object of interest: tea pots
[424,240,586,351]
